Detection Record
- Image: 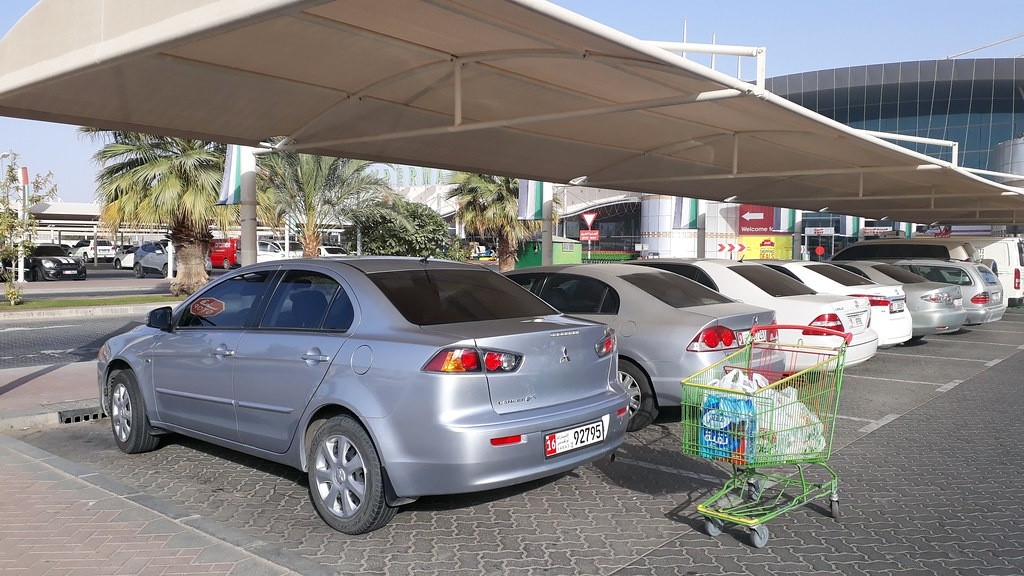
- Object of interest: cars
[622,256,880,375]
[819,259,966,345]
[98,255,633,533]
[502,261,787,432]
[746,258,914,349]
[886,259,1005,326]
[1,230,353,286]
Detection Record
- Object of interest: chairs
[275,291,343,328]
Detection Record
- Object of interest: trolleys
[680,324,856,549]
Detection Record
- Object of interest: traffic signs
[738,204,775,236]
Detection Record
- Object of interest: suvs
[919,234,1024,307]
[826,240,998,280]
